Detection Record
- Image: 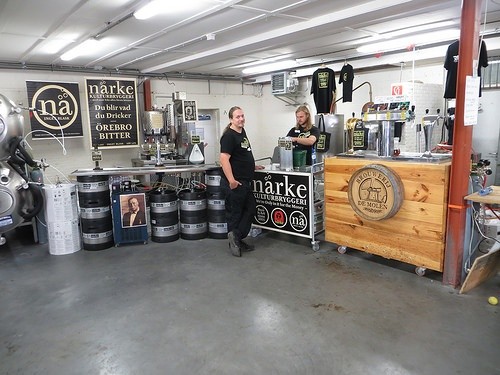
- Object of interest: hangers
[319,59,328,69]
[343,59,349,66]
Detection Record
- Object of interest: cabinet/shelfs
[324,149,473,277]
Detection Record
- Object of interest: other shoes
[228,232,242,257]
[229,241,254,251]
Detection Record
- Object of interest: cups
[394,148,400,156]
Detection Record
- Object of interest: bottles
[479,187,493,195]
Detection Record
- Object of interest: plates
[430,152,452,158]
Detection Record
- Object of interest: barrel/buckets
[77,168,229,251]
[294,147,307,166]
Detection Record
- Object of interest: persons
[219,106,256,257]
[286,106,320,166]
[123,198,146,226]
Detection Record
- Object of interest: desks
[464,185,500,274]
